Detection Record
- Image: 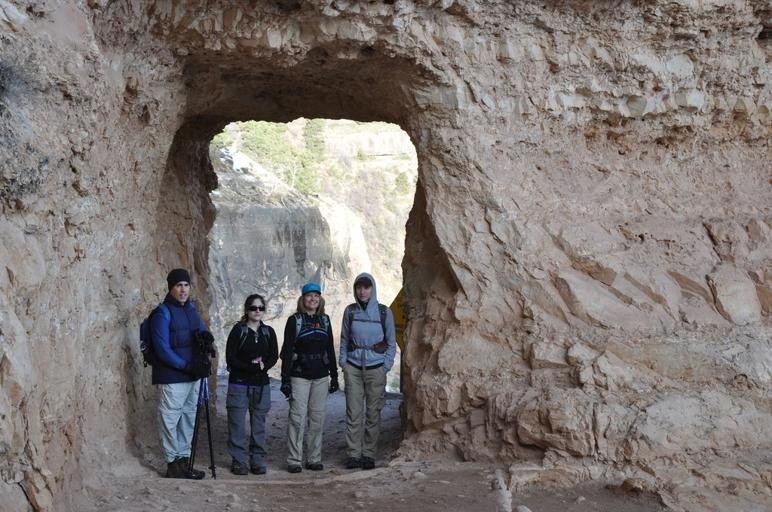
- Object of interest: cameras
[193,339,216,357]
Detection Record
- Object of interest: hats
[168,269,190,290]
[302,283,321,296]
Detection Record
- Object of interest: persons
[224,294,279,478]
[339,271,396,470]
[147,267,215,481]
[280,282,339,474]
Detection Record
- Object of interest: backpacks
[140,302,196,365]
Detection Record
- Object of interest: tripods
[188,357,216,480]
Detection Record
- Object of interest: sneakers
[167,457,375,479]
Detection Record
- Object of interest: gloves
[280,383,291,397]
[329,378,338,393]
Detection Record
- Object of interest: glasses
[247,305,265,312]
